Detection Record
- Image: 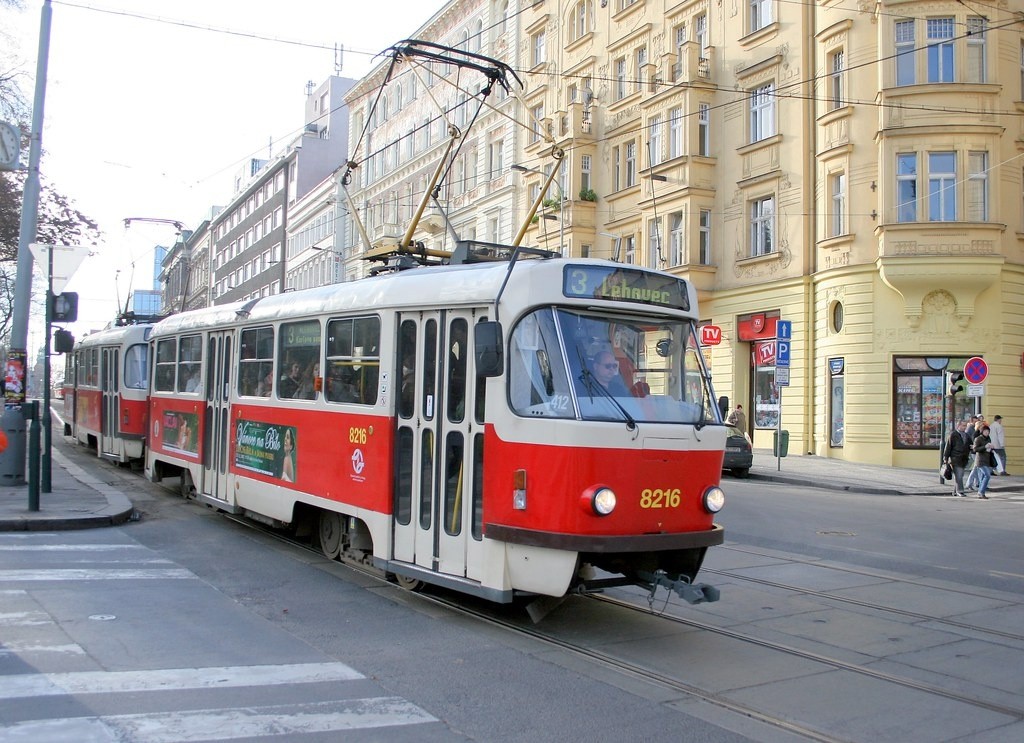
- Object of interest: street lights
[511,164,564,258]
[312,247,346,283]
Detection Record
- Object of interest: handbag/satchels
[939,462,953,480]
[992,450,1003,472]
[990,451,997,467]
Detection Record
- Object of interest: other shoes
[1001,471,1009,476]
[967,487,974,490]
[991,472,997,476]
[953,493,966,497]
[979,495,988,499]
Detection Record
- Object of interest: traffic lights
[946,372,964,396]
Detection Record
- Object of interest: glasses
[599,363,617,369]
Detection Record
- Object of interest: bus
[141,39,731,625]
[54,378,66,400]
[62,243,192,471]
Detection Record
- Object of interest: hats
[994,415,1002,420]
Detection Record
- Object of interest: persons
[691,381,699,403]
[448,393,466,478]
[728,404,747,436]
[990,415,1010,476]
[973,425,992,499]
[581,351,628,397]
[166,330,485,417]
[281,428,295,482]
[965,413,989,490]
[943,419,974,497]
[176,414,191,451]
